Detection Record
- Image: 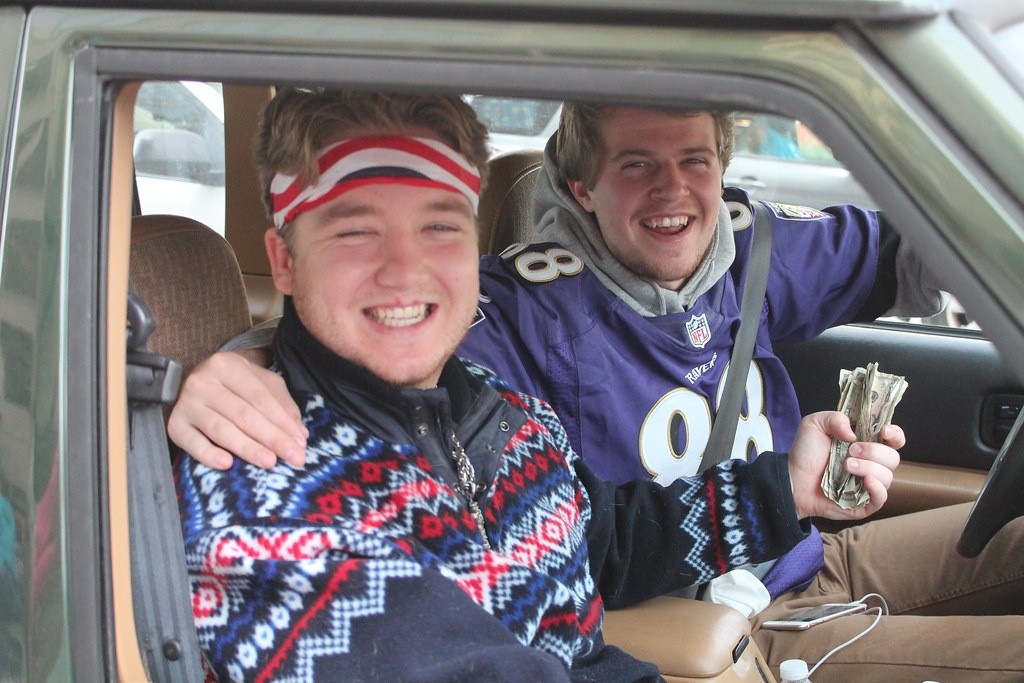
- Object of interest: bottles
[776,658,813,683]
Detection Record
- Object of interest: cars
[1,0,1024,682]
[129,70,991,327]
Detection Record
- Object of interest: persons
[172,83,669,683]
[447,97,1024,682]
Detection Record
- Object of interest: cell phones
[763,604,868,631]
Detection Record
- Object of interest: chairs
[127,214,252,422]
[475,151,544,255]
